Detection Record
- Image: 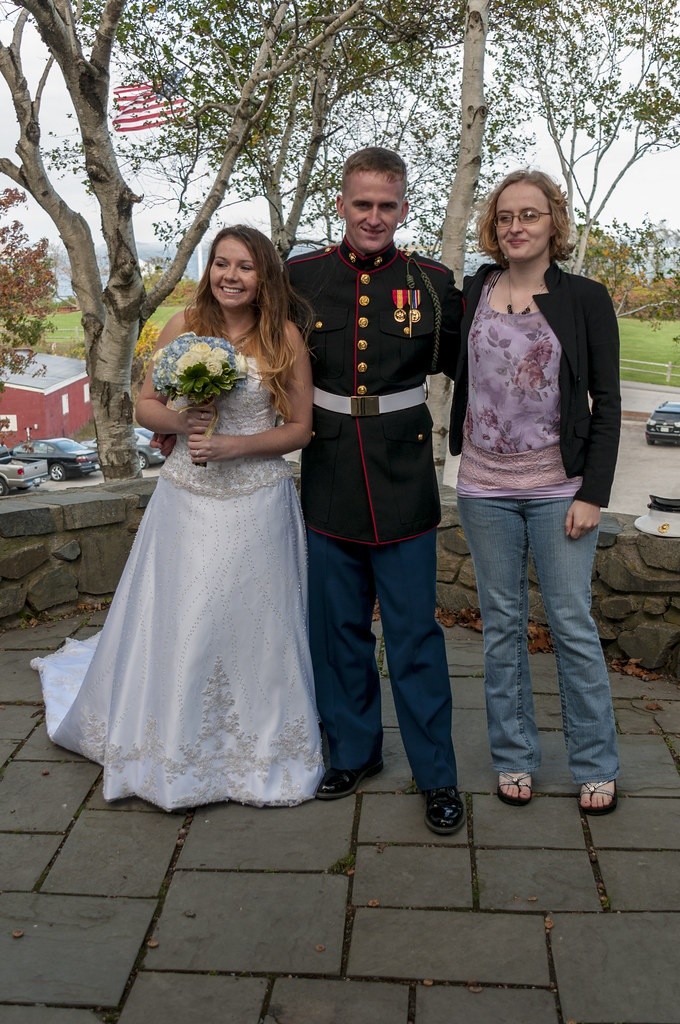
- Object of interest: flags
[113,66,191,132]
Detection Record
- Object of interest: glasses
[494,209,551,227]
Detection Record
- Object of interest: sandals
[497,770,532,806]
[579,778,617,816]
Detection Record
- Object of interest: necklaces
[506,266,546,315]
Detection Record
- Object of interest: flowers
[150,333,250,406]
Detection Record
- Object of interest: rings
[196,450,200,457]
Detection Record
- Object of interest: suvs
[645,401,680,446]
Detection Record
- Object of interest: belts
[312,385,425,416]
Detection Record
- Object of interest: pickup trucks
[0,444,48,498]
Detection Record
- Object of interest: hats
[634,495,680,537]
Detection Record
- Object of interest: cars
[9,437,101,481]
[79,427,167,470]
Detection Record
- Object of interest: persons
[448,171,622,815]
[30,223,326,812]
[149,146,466,834]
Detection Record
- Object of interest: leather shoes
[422,786,465,834]
[315,757,383,799]
